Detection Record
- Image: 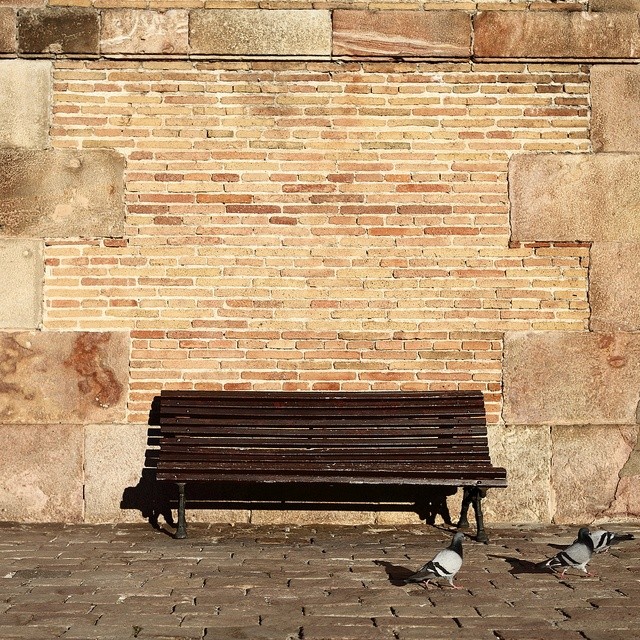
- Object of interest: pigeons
[530,526,594,580]
[587,529,634,554]
[400,532,470,590]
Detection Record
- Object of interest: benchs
[153,389,508,540]
